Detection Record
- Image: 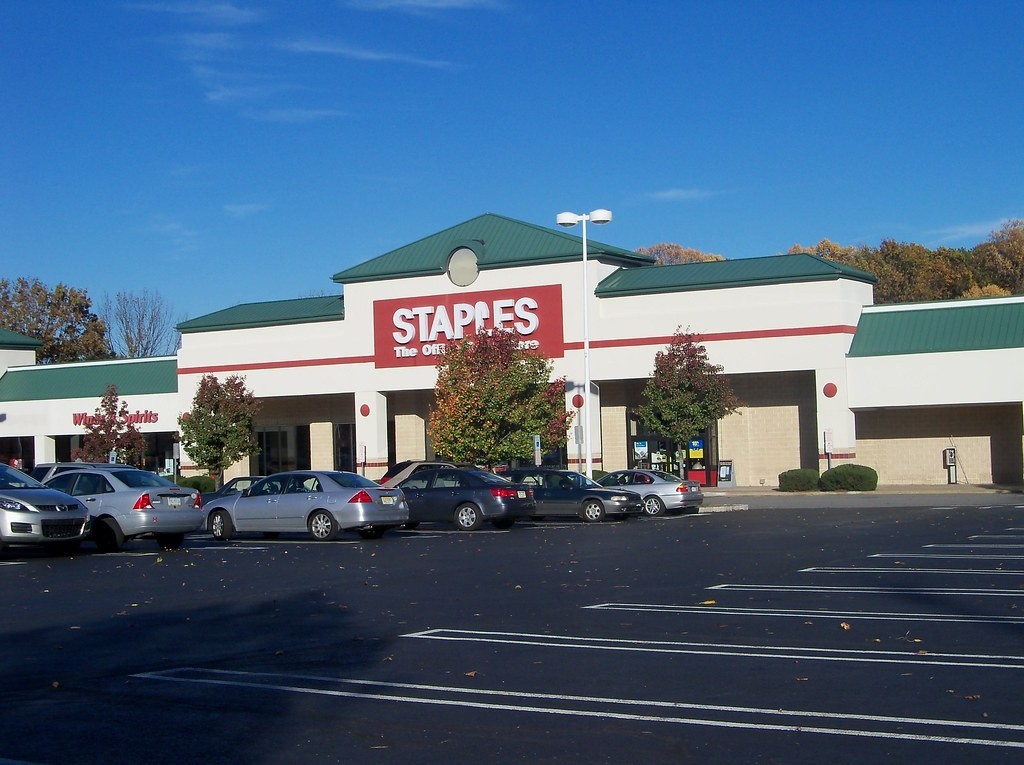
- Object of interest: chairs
[433,478,445,488]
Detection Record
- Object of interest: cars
[0,463,91,560]
[393,467,538,532]
[594,468,704,517]
[379,460,462,490]
[201,470,411,541]
[43,467,204,554]
[200,475,313,509]
[496,468,645,523]
[29,462,163,496]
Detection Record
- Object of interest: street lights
[555,209,612,487]
